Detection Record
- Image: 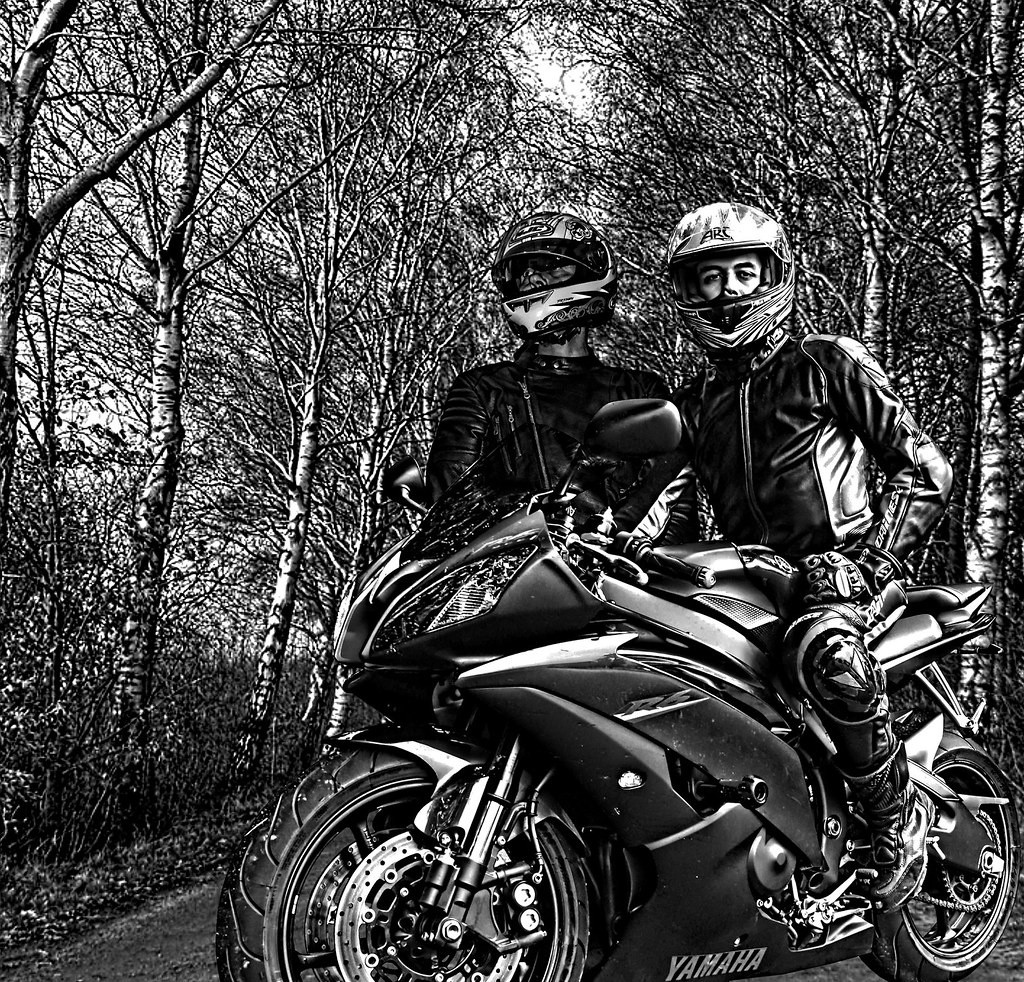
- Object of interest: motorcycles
[214,401,1018,982]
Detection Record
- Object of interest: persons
[429,213,701,544]
[667,202,954,912]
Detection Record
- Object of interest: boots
[838,737,937,916]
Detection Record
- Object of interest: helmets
[490,212,617,348]
[666,201,797,354]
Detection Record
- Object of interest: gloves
[796,542,904,606]
